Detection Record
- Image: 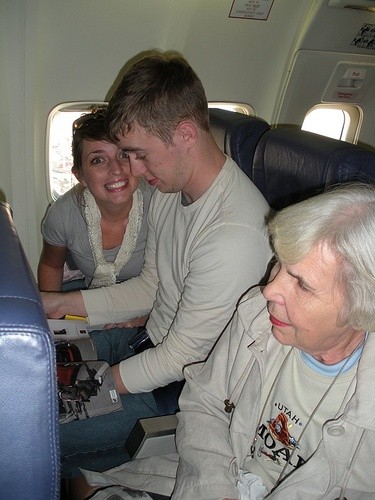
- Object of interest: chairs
[0,201,62,500]
[206,107,375,212]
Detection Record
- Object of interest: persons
[36,106,159,334]
[77,183,374,500]
[35,50,279,491]
